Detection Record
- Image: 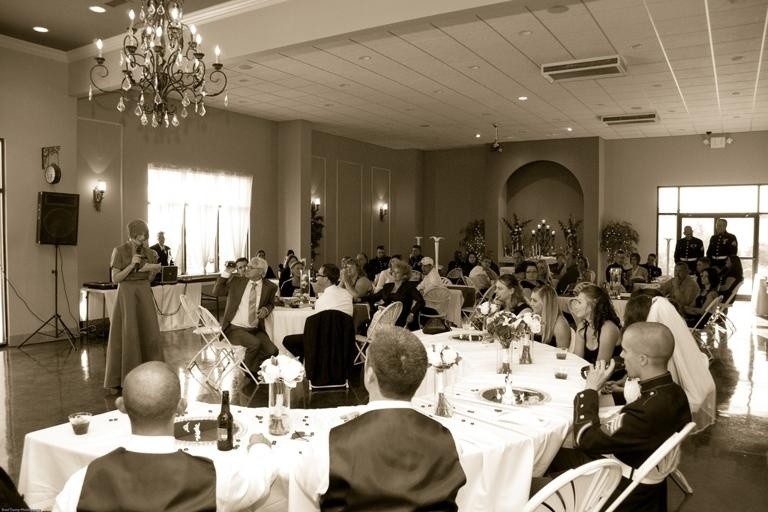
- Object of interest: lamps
[304,309,355,390]
[687,295,724,361]
[354,301,404,365]
[520,458,622,512]
[605,422,699,512]
[418,286,451,329]
[708,280,745,334]
[446,267,461,285]
[180,294,223,369]
[461,283,498,330]
[462,275,487,302]
[195,305,260,391]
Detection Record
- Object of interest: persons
[485,250,500,277]
[469,255,496,304]
[212,257,279,384]
[374,257,400,307]
[576,256,596,284]
[640,254,662,279]
[681,267,718,329]
[529,321,692,512]
[149,232,174,266]
[279,255,298,288]
[337,257,377,302]
[718,255,742,304]
[550,253,567,276]
[520,262,546,290]
[626,253,649,293]
[531,284,570,348]
[556,254,580,296]
[574,285,626,406]
[706,219,738,274]
[369,245,391,281]
[103,218,165,395]
[408,245,423,273]
[416,257,444,297]
[696,257,710,292]
[294,325,467,512]
[536,259,554,285]
[606,251,627,290]
[282,263,353,364]
[254,248,276,279]
[674,226,704,275]
[51,360,278,512]
[463,252,479,277]
[356,252,369,278]
[601,288,716,436]
[283,250,294,269]
[512,250,536,280]
[493,274,534,317]
[353,260,425,328]
[280,261,315,297]
[671,262,700,307]
[236,257,250,274]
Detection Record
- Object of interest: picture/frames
[77,283,202,343]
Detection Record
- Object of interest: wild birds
[155,265,178,285]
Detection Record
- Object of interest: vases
[478,302,498,342]
[425,342,463,415]
[517,311,541,363]
[489,311,523,372]
[256,353,302,433]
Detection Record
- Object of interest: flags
[483,316,494,344]
[266,379,290,435]
[430,366,455,417]
[496,342,514,373]
[520,334,535,363]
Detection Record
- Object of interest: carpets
[248,282,256,326]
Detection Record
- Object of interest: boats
[36,191,80,247]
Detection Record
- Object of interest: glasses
[312,197,320,215]
[89,0,229,128]
[380,204,387,220]
[93,180,105,205]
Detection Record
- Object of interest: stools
[710,256,728,259]
[680,258,698,261]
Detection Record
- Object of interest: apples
[538,225,541,231]
[531,229,535,236]
[546,225,550,231]
[516,242,520,251]
[512,245,515,257]
[520,244,524,252]
[538,244,541,259]
[531,245,535,258]
[542,220,546,226]
[535,243,538,259]
[551,230,556,238]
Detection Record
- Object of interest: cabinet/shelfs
[217,391,233,451]
[582,362,625,378]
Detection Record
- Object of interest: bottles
[417,255,434,267]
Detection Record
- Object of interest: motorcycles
[17,245,79,353]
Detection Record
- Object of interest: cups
[315,272,329,278]
[244,265,264,271]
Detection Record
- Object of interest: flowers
[135,247,142,273]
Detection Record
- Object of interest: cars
[582,357,626,381]
[68,411,91,434]
[554,365,567,380]
[555,347,568,360]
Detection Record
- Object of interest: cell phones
[224,260,237,268]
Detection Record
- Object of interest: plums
[488,143,503,153]
